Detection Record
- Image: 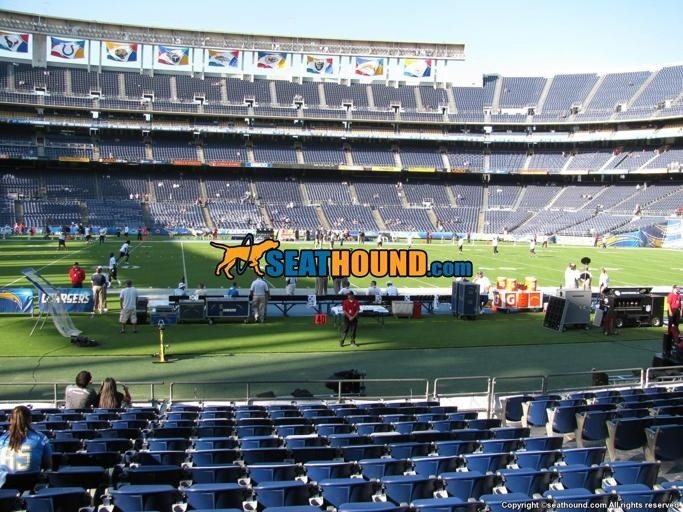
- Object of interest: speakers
[645,294,665,327]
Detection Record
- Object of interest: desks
[538,286,675,331]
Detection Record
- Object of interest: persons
[86,377,131,410]
[65,371,97,409]
[1,220,608,296]
[0,406,52,473]
[339,291,360,347]
[118,280,140,333]
[666,283,682,337]
[248,275,269,322]
